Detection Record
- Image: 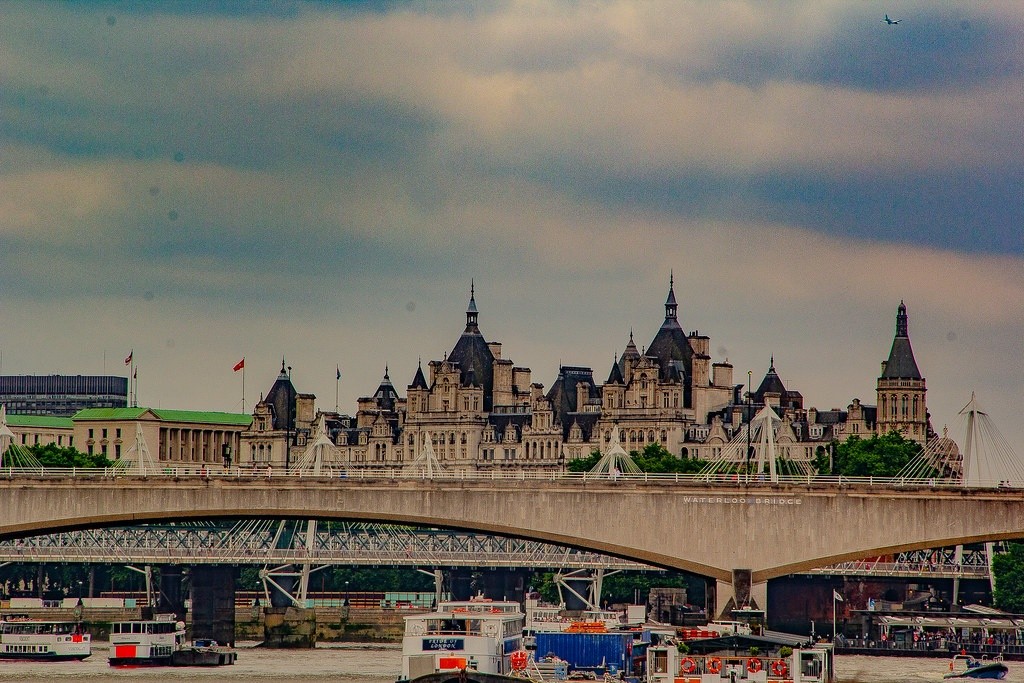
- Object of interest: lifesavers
[452,608,470,612]
[510,651,528,670]
[681,658,695,672]
[747,657,761,672]
[771,659,788,674]
[708,657,721,671]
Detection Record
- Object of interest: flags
[125,351,132,366]
[233,359,244,371]
[134,368,137,379]
[337,369,341,379]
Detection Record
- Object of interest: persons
[221,461,347,480]
[608,466,620,479]
[917,629,1016,668]
[929,465,963,489]
[998,479,1012,488]
[712,466,774,485]
[200,464,209,477]
[2,622,109,641]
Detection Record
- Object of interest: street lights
[78,581,83,606]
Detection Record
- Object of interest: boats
[108,614,186,666]
[943,653,1008,679]
[0,621,91,660]
[394,587,835,683]
[173,637,237,666]
[810,610,1024,662]
